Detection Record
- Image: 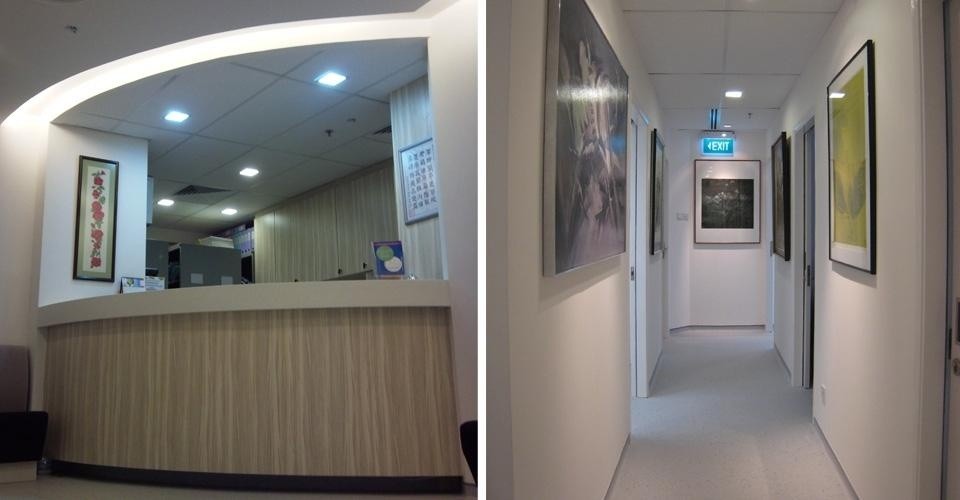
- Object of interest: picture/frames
[692,159,762,246]
[648,128,667,256]
[72,154,120,281]
[399,135,436,226]
[827,38,877,278]
[771,129,790,261]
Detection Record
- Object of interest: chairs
[1,344,49,485]
